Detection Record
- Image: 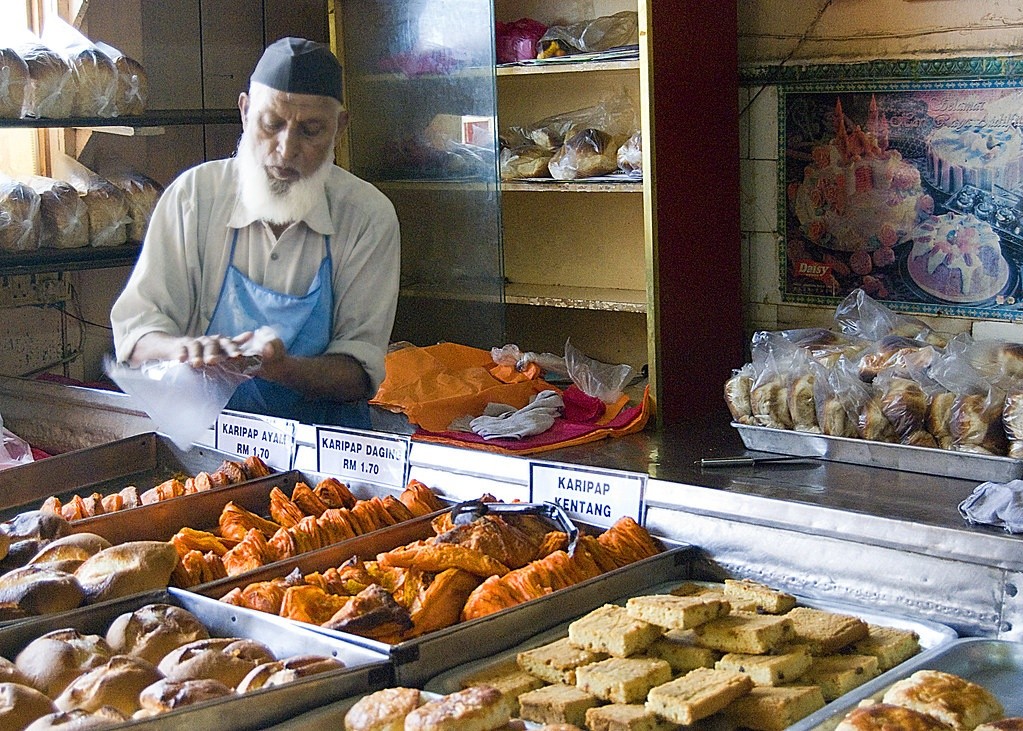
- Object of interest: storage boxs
[0,429,1023,731]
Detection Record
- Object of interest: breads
[0,457,1023,731]
[0,171,167,249]
[723,317,1023,460]
[377,123,623,178]
[0,47,149,118]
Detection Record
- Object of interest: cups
[995,207,1017,231]
[956,192,973,213]
[973,201,995,221]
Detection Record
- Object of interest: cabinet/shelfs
[323,0,743,431]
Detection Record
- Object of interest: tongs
[451,501,579,558]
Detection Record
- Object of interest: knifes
[702,456,821,468]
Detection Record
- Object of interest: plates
[794,213,925,253]
[921,155,1023,196]
[898,240,1020,309]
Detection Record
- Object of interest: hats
[251,37,344,105]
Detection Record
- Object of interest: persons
[110,36,401,430]
